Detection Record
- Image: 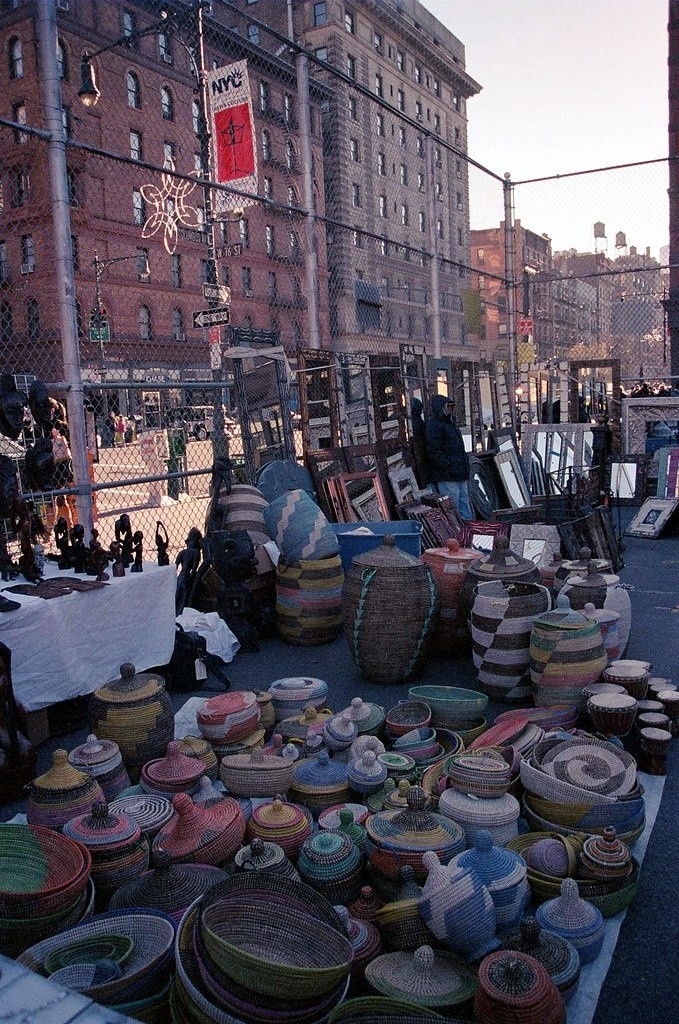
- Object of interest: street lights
[77,1,231,496]
[93,251,151,447]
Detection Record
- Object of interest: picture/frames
[620,397,679,484]
[395,492,463,550]
[624,495,679,539]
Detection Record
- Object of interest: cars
[0,432,28,485]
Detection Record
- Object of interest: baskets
[168,871,355,1024]
[506,734,646,917]
[386,685,489,815]
[0,822,178,1024]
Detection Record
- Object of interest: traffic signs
[192,306,231,331]
[202,282,231,305]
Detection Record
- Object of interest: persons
[9,493,202,617]
[105,410,126,448]
[578,396,589,423]
[1,374,73,540]
[210,527,262,654]
[423,395,472,520]
[620,381,678,399]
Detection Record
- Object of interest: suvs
[167,405,237,443]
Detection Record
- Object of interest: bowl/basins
[0,686,648,1024]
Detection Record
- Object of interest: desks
[0,558,178,747]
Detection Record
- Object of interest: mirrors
[493,451,530,509]
[657,446,679,501]
[335,472,391,522]
[343,445,392,501]
[226,325,622,486]
[388,467,420,503]
[520,423,600,495]
[461,495,625,573]
[603,454,648,506]
[308,448,349,521]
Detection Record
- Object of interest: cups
[638,677,679,757]
